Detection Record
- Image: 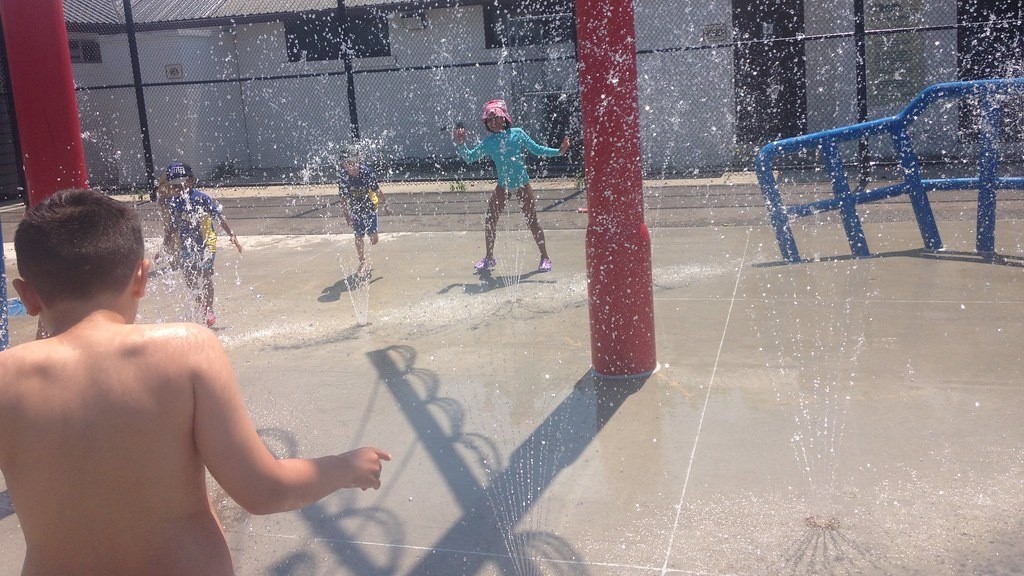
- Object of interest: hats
[337,146,361,165]
[165,162,193,185]
[482,99,512,126]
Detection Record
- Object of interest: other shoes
[539,259,551,271]
[206,313,215,326]
[474,257,498,271]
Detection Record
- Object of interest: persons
[0,189,390,576]
[339,146,392,274]
[154,159,243,327]
[453,99,570,271]
